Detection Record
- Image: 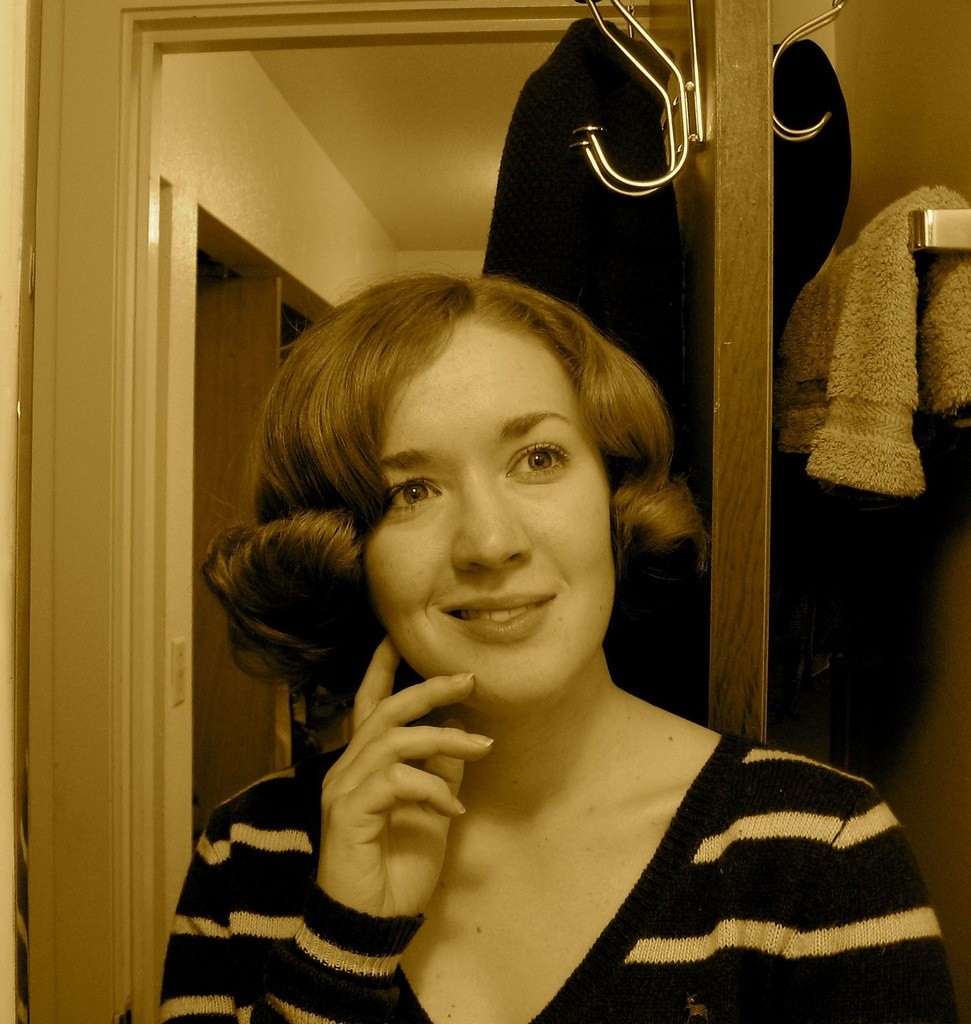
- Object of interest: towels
[773,184,970,497]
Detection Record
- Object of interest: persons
[157,275,958,1024]
